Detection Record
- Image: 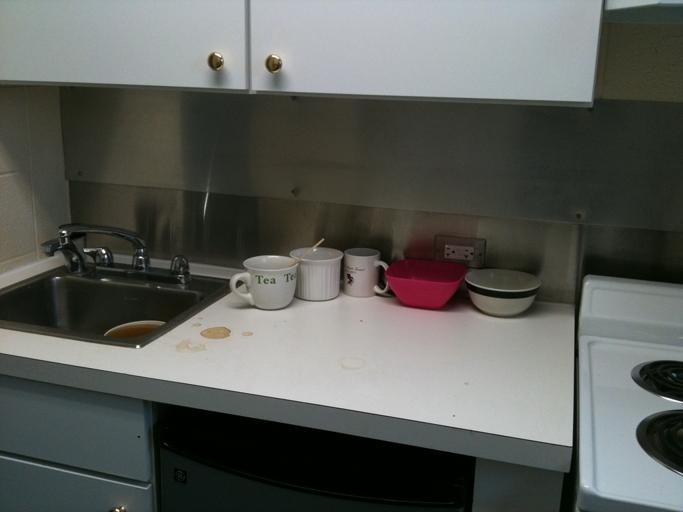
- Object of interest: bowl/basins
[464,263,543,318]
[103,321,174,346]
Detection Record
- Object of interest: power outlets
[433,234,485,268]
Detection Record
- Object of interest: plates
[385,258,468,309]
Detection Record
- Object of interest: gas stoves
[578,274,683,512]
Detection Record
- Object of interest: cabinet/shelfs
[0,1,605,107]
[0,372,156,512]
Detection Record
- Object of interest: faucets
[39,240,88,275]
[56,221,146,271]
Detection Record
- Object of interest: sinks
[0,262,243,349]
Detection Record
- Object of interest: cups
[228,255,299,309]
[290,247,345,301]
[345,246,391,298]
